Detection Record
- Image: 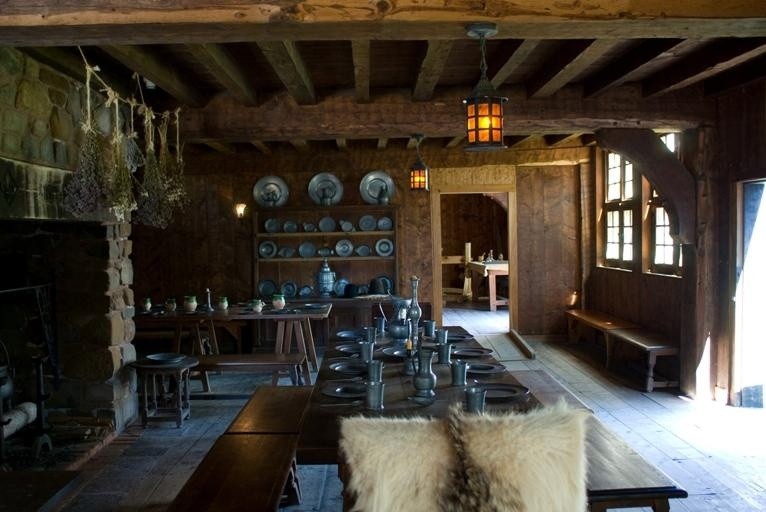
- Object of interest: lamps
[457,17,512,151]
[405,130,432,192]
[235,202,247,227]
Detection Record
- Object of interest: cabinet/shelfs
[248,202,404,355]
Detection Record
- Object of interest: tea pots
[375,288,439,406]
[316,262,337,292]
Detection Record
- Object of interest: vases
[408,275,422,357]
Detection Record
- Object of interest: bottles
[137,292,286,314]
[408,275,423,337]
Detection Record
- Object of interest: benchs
[543,410,690,511]
[161,429,308,512]
[131,316,249,355]
[561,305,643,375]
[505,365,596,417]
[130,347,309,400]
[220,381,315,504]
[601,325,683,393]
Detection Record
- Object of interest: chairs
[333,400,595,512]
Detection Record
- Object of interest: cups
[359,316,388,409]
[424,318,488,414]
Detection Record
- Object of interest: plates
[258,237,397,259]
[323,324,366,398]
[146,351,187,363]
[251,171,396,205]
[296,303,329,310]
[265,215,395,233]
[255,275,394,296]
[444,331,531,400]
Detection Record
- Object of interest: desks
[126,352,200,430]
[464,256,508,313]
